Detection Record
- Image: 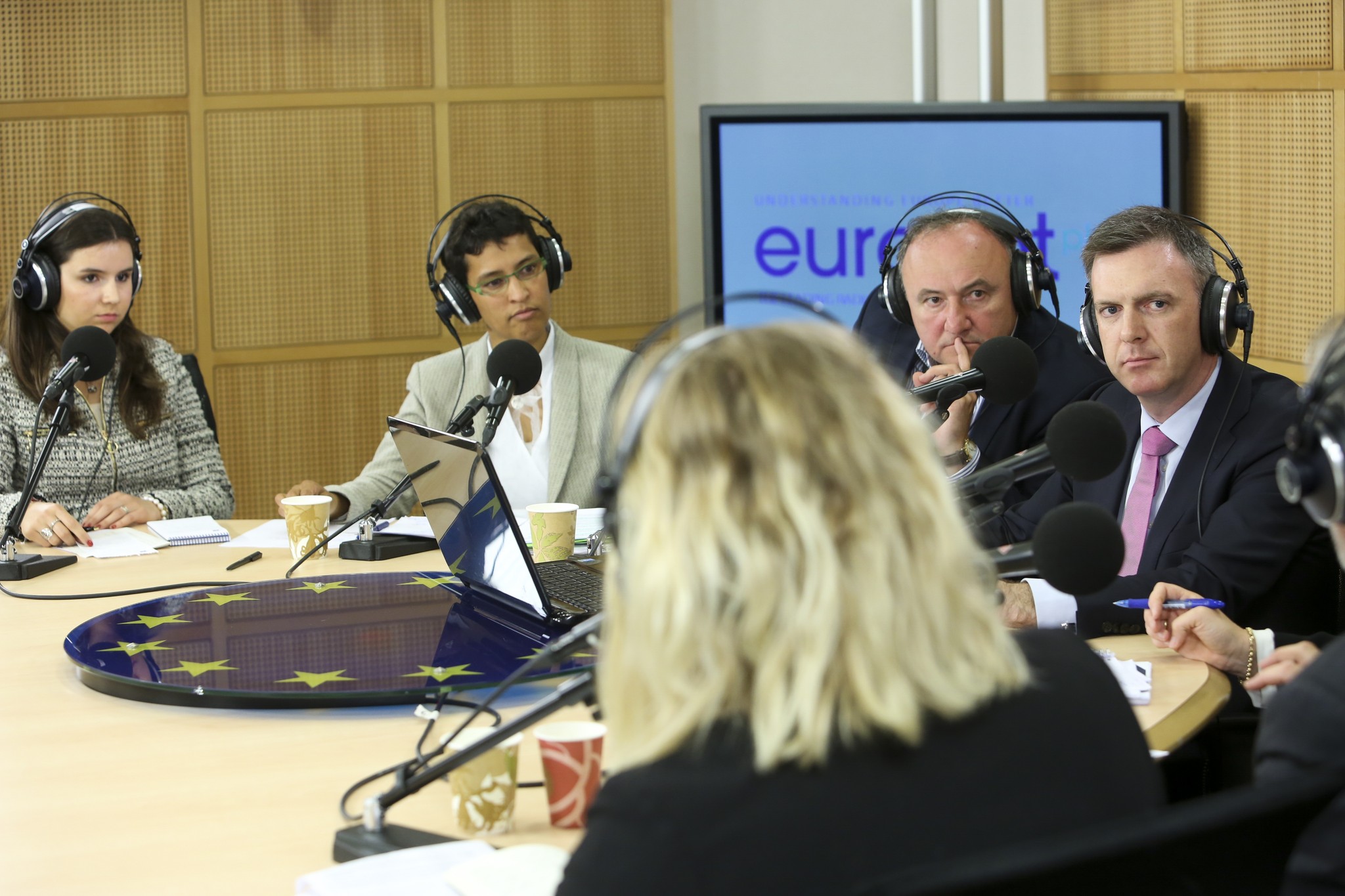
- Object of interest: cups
[526,503,579,563]
[440,726,523,836]
[534,721,607,828]
[281,495,332,560]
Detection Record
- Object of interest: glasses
[465,256,547,296]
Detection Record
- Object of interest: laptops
[383,415,609,633]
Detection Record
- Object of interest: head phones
[1079,215,1252,357]
[12,192,143,313]
[1273,316,1345,529]
[879,190,1052,326]
[426,194,571,326]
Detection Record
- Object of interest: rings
[50,520,60,531]
[40,528,54,541]
[120,506,128,513]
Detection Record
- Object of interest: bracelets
[142,494,168,522]
[1239,628,1255,682]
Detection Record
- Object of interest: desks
[0,520,1233,896]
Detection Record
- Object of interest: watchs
[937,438,977,467]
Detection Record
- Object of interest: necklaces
[84,380,97,392]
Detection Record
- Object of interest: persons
[1001,203,1341,806]
[0,190,237,549]
[273,193,644,524]
[1252,315,1345,896]
[557,322,1164,896]
[853,190,1105,576]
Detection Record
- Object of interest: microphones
[482,338,543,446]
[42,324,115,402]
[950,400,1129,499]
[908,337,1037,405]
[990,503,1125,596]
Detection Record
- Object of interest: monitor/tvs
[694,101,1186,357]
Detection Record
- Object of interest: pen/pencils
[1112,598,1225,609]
[226,551,263,570]
[83,525,99,531]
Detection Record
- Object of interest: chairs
[833,767,1345,896]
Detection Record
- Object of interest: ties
[1119,427,1178,577]
